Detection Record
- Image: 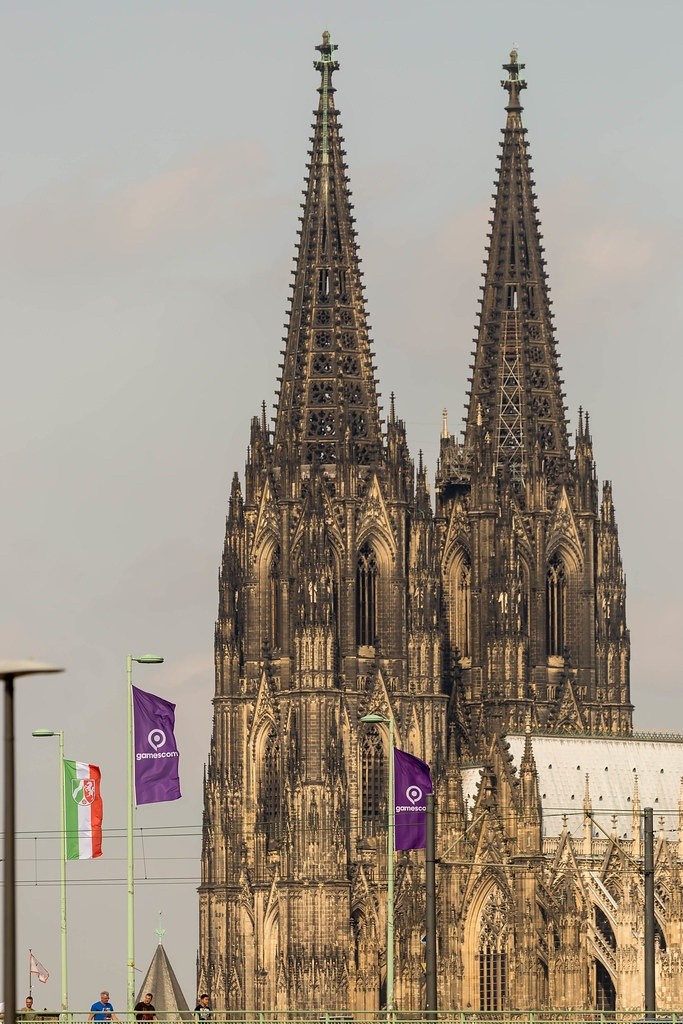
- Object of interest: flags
[30,955,50,983]
[393,748,431,854]
[131,686,182,804]
[62,761,105,860]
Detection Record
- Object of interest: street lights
[125,653,165,1024]
[1,657,65,1024]
[361,714,395,1024]
[32,729,69,1022]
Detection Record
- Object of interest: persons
[193,995,212,1024]
[88,990,122,1024]
[0,997,39,1024]
[133,992,158,1024]
